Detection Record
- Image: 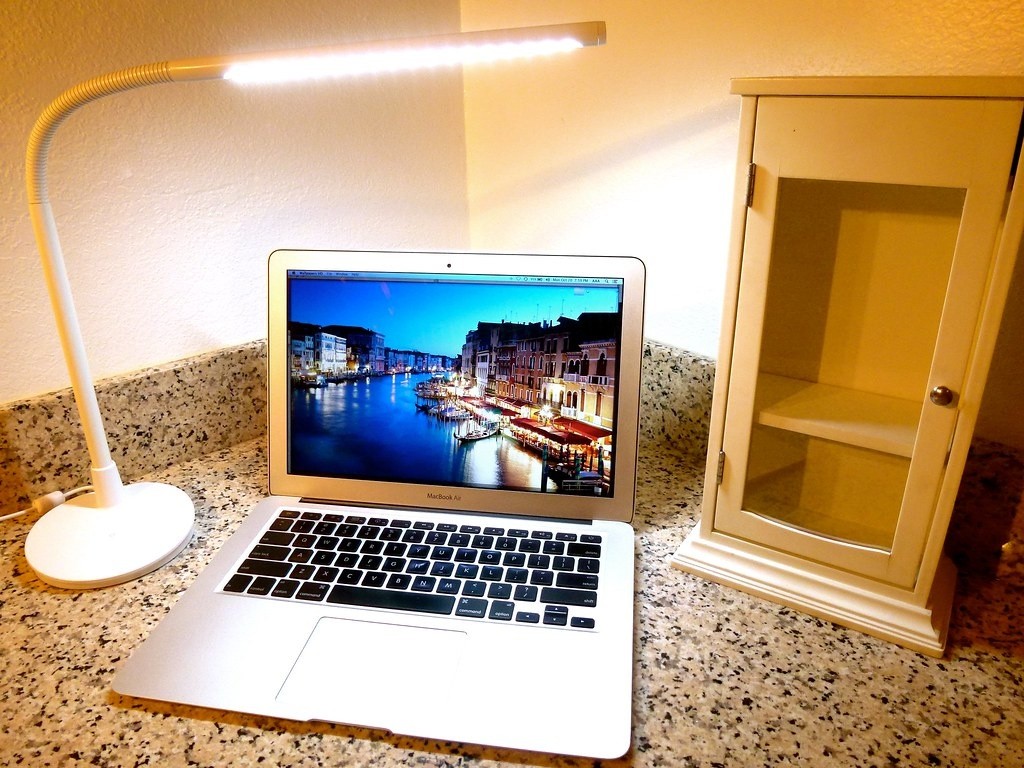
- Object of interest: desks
[0,419,1024,768]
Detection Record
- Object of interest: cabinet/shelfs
[669,75,1024,660]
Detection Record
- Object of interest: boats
[413,374,474,420]
[453,411,502,442]
[305,375,328,388]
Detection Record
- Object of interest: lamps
[22,15,608,592]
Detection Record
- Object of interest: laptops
[113,250,648,759]
